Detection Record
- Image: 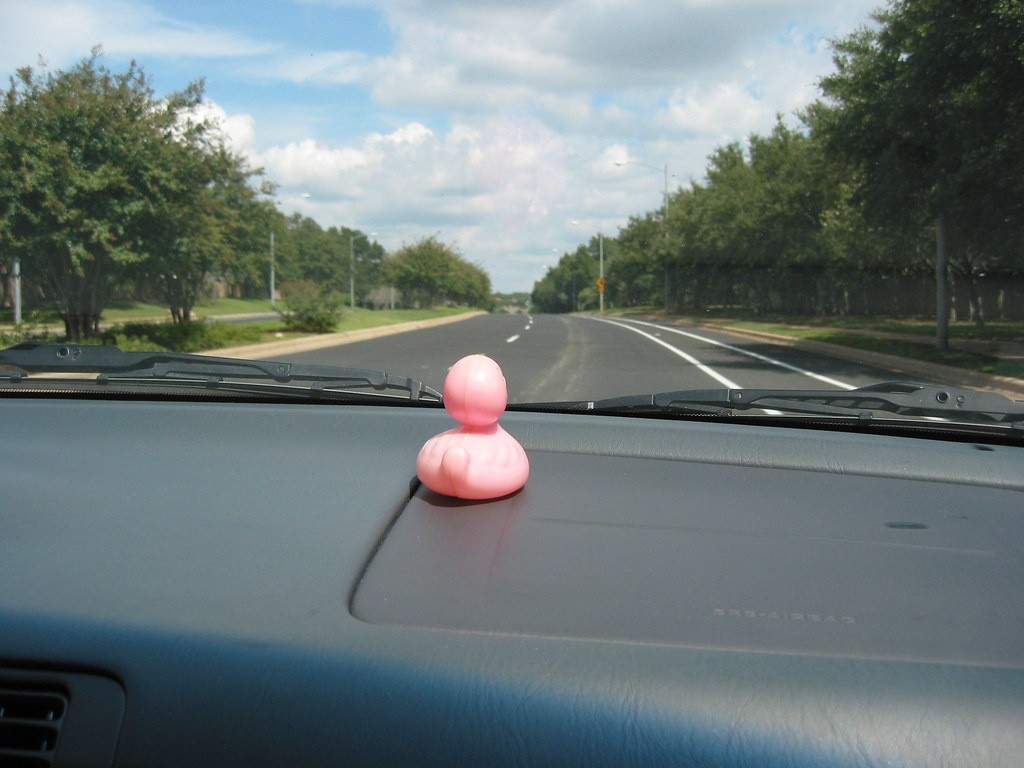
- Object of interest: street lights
[614,158,671,315]
[269,192,312,306]
[349,232,378,309]
[570,220,605,313]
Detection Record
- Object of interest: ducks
[415,353,529,500]
